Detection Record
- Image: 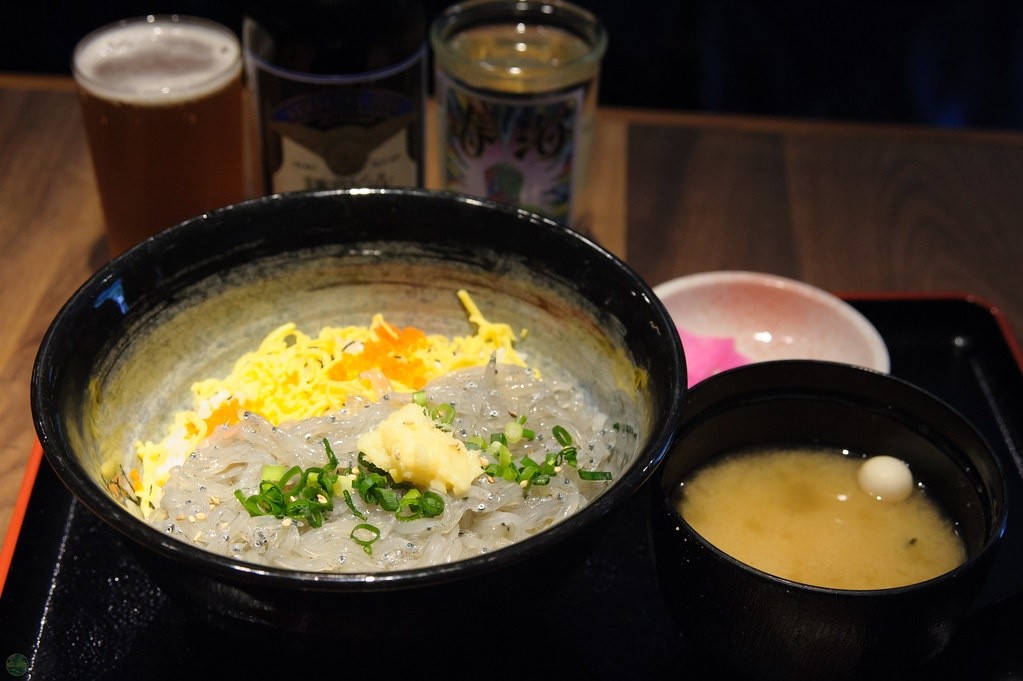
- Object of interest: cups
[70,18,262,258]
[431,0,607,229]
[227,0,431,193]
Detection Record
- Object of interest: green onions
[233,391,613,557]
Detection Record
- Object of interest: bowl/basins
[30,191,686,631]
[657,360,1010,681]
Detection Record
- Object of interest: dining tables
[0,73,1023,681]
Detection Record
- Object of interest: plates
[654,272,889,388]
